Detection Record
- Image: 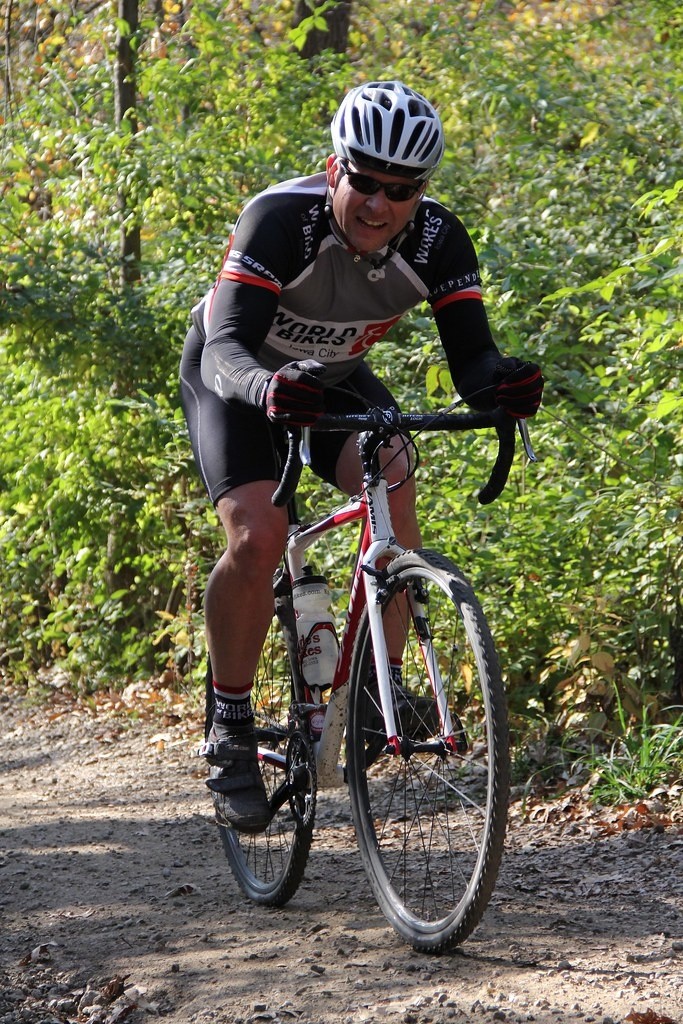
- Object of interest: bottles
[292,565,340,686]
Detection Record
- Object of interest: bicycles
[204,374,538,954]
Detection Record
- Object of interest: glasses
[339,160,424,202]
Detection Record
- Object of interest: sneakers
[204,726,269,834]
[365,683,439,747]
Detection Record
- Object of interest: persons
[179,80,544,834]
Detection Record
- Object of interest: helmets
[331,81,445,183]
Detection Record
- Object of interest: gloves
[259,359,326,429]
[493,357,545,420]
